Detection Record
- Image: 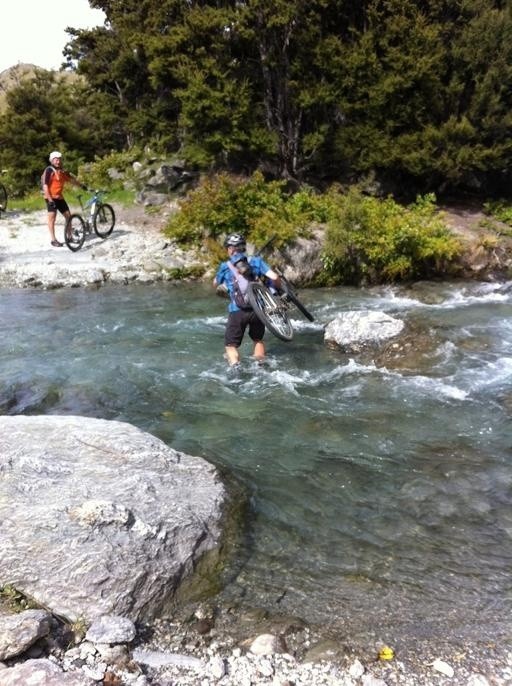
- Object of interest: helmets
[49,151,61,162]
[223,232,246,247]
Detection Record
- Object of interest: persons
[40,150,90,248]
[211,230,279,369]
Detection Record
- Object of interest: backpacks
[227,255,258,310]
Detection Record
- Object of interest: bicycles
[243,233,314,342]
[64,188,116,254]
[0,170,8,213]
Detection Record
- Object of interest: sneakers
[51,240,63,246]
[67,238,77,242]
